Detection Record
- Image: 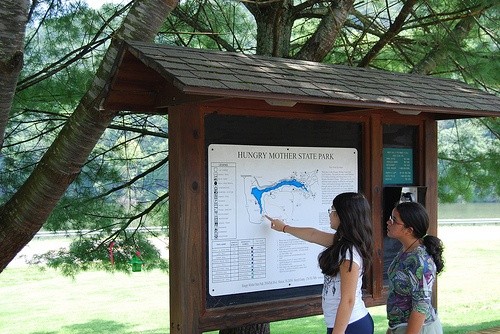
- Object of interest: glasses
[328,209,337,214]
[389,216,406,225]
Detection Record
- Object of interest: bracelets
[283,225,289,233]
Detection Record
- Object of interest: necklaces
[394,239,419,261]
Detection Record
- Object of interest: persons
[265,192,374,334]
[386,202,446,334]
